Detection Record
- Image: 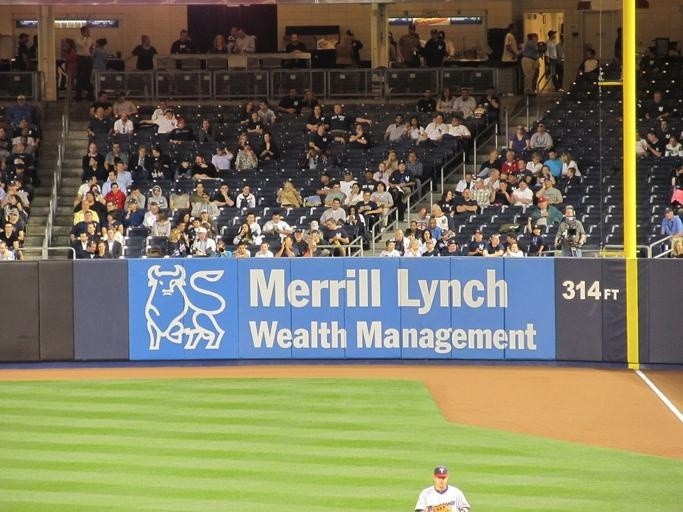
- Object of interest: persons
[636,86,681,257]
[64,24,624,260]
[0,34,41,261]
[413,465,471,512]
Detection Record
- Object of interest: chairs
[1,57,683,260]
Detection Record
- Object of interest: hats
[434,467,447,478]
